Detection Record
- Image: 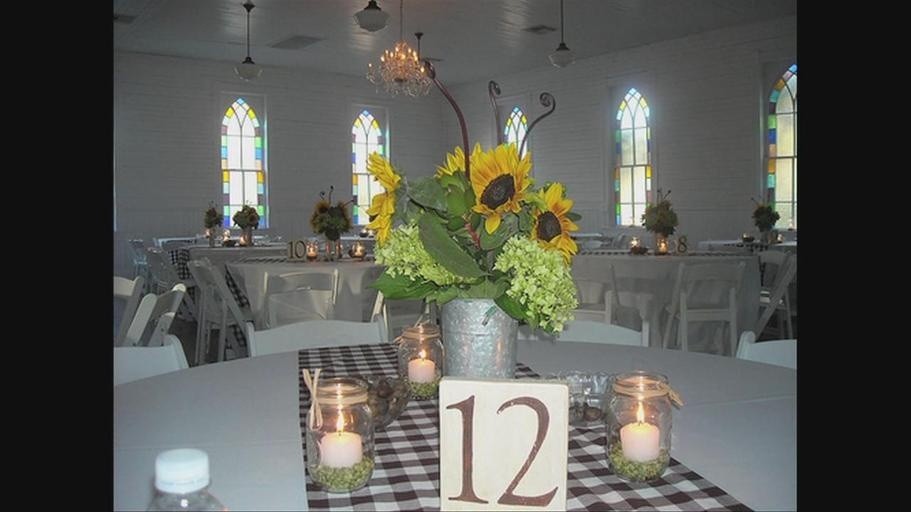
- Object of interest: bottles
[305,240,318,261]
[143,449,225,512]
[604,374,673,484]
[350,240,365,258]
[306,381,376,492]
[395,325,446,401]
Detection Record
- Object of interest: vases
[655,232,668,255]
[240,225,251,246]
[759,226,771,245]
[441,300,517,382]
[325,232,340,258]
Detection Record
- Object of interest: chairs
[114,238,797,366]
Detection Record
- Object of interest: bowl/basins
[538,372,633,432]
[322,376,410,428]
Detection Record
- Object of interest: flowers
[368,58,580,337]
[751,190,781,232]
[232,204,260,230]
[310,186,359,240]
[642,190,678,236]
[204,208,223,228]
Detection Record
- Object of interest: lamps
[547,0,577,68]
[366,1,433,98]
[234,0,263,79]
[353,0,390,33]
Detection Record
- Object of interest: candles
[209,227,217,247]
[620,401,659,462]
[320,413,363,469]
[408,349,435,384]
